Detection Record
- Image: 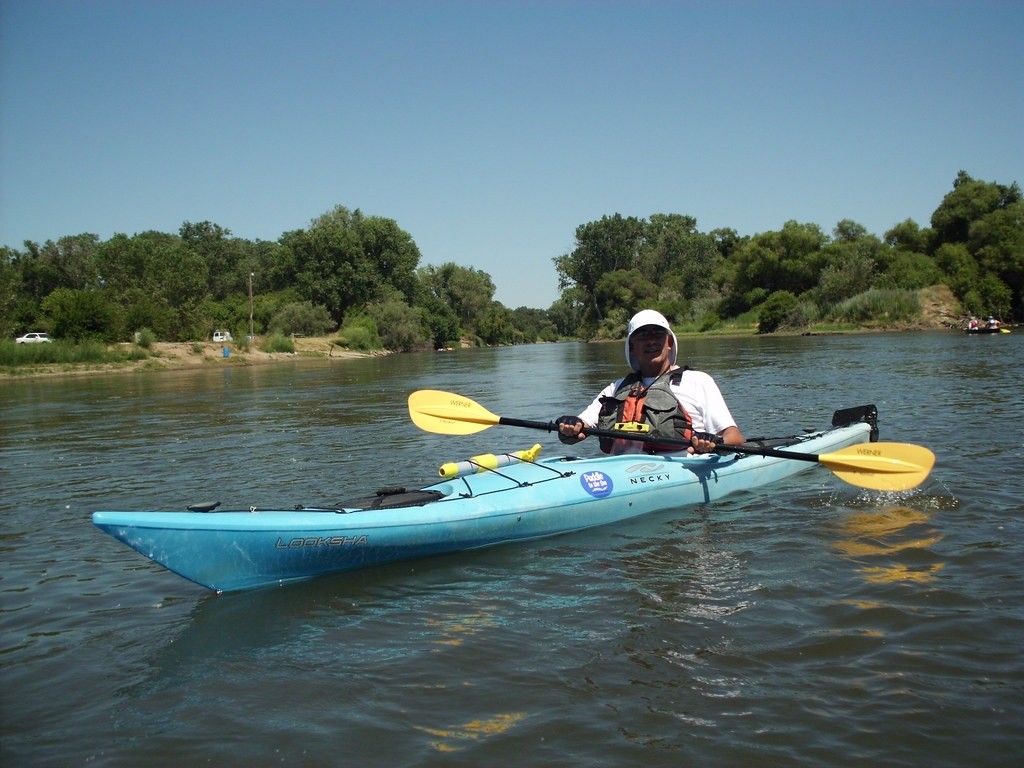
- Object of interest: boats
[92,404,881,594]
[961,328,1000,334]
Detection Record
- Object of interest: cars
[212,331,233,342]
[16,332,56,344]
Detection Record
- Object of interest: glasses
[636,329,665,339]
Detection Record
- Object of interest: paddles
[406,387,938,493]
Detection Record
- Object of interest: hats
[624,308,679,371]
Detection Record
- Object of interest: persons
[984,316,1000,329]
[554,309,744,454]
[963,319,978,330]
